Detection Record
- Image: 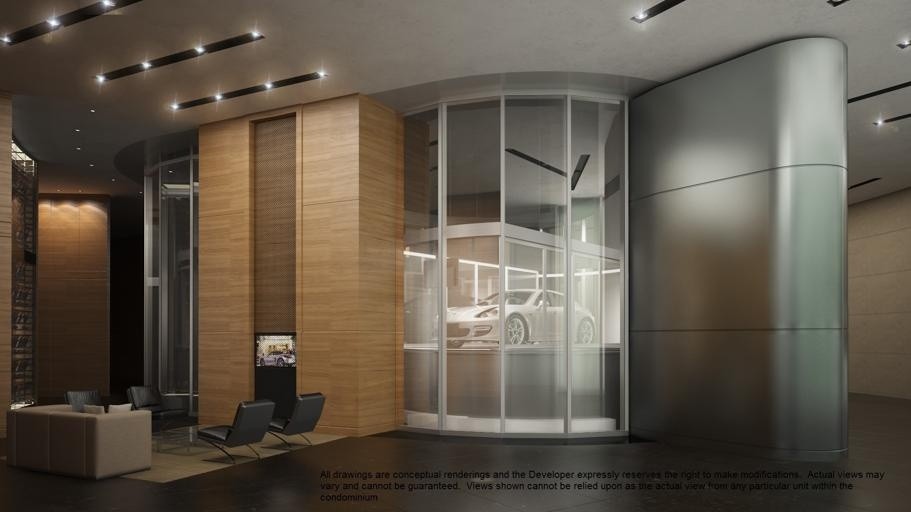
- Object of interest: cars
[443,286,600,347]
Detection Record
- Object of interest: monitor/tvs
[254,332,295,369]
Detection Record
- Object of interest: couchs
[11,403,151,479]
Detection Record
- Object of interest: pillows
[83,403,136,419]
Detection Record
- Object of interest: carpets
[116,423,344,484]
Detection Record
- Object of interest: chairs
[195,392,322,468]
[65,390,100,406]
[126,386,188,425]
